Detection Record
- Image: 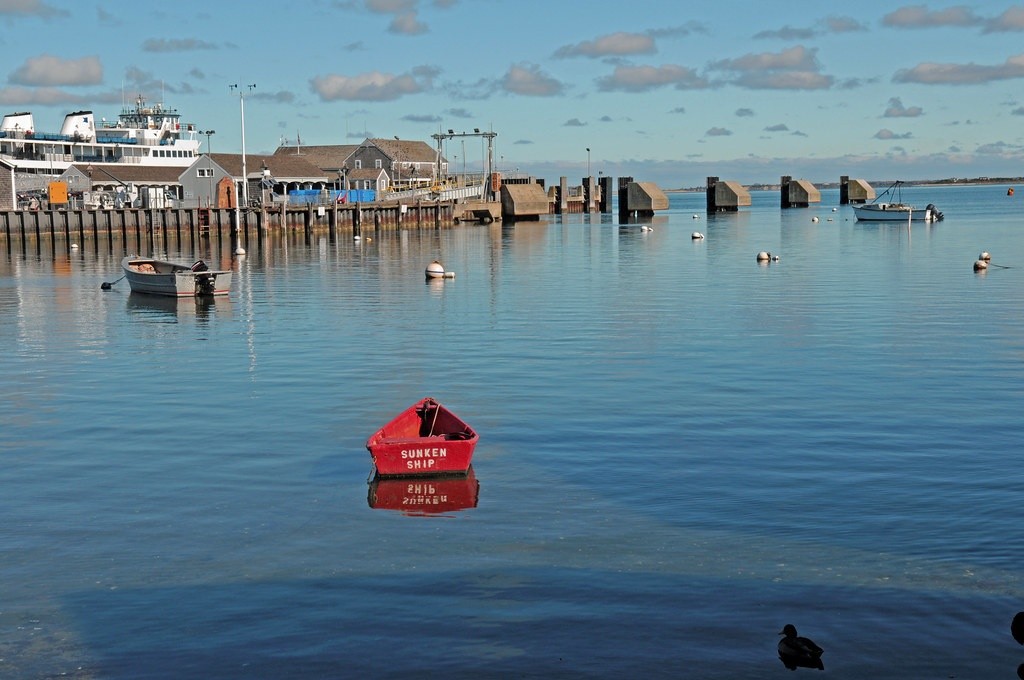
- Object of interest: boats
[366,462,479,515]
[121,253,234,297]
[365,398,479,476]
[852,180,944,223]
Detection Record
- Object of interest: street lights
[586,148,590,177]
[454,155,459,185]
[393,135,401,192]
[230,83,257,205]
[461,139,466,186]
[197,129,215,207]
